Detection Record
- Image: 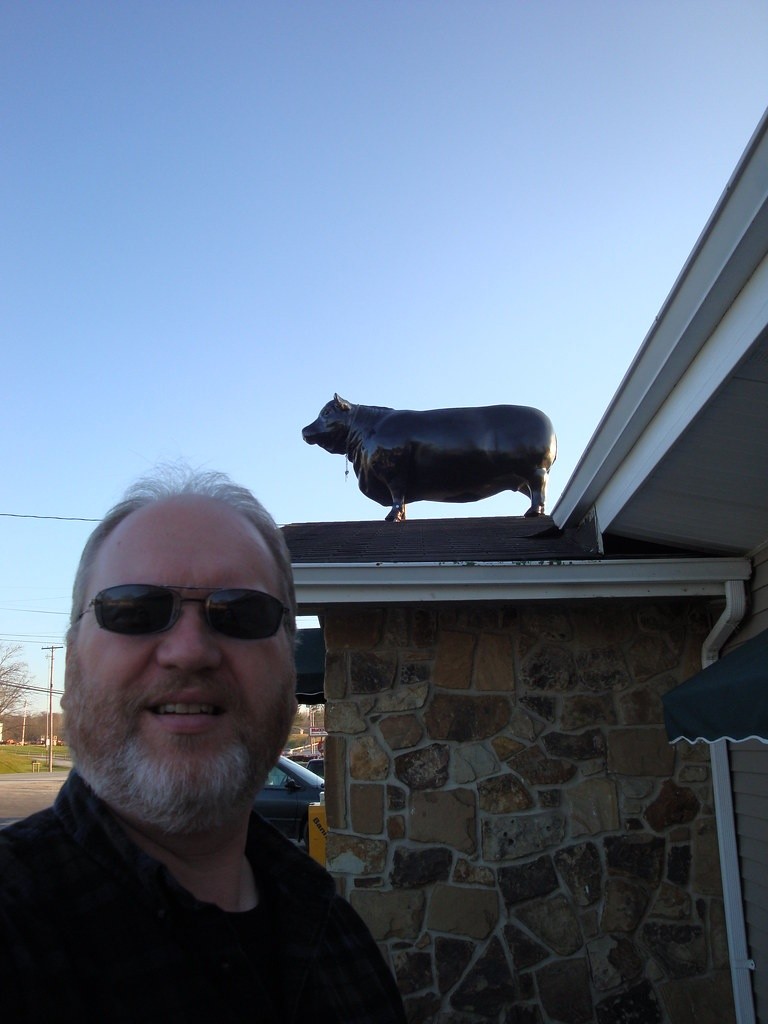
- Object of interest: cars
[0,737,45,745]
[286,754,326,782]
[253,754,327,845]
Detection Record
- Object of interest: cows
[301,392,557,520]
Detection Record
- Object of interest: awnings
[662,626,768,747]
[292,627,325,706]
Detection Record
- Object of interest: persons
[1,470,406,1024]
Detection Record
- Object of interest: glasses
[76,583,295,639]
